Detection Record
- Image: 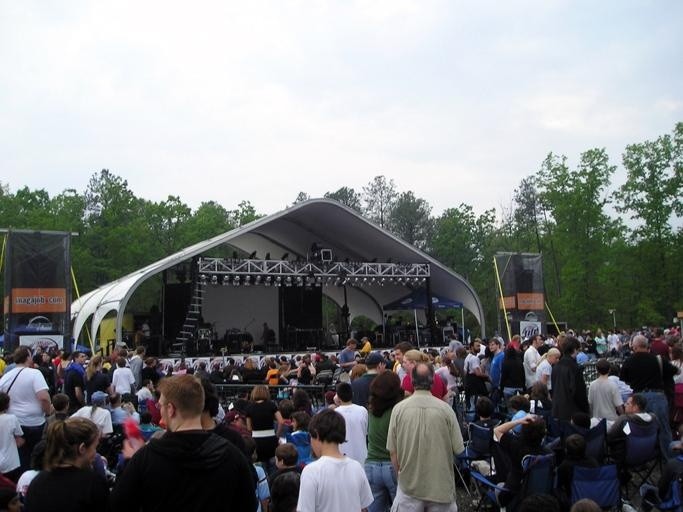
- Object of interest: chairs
[470,455,556,512]
[467,420,502,455]
[486,439,522,485]
[560,419,612,465]
[620,418,665,502]
[570,464,619,508]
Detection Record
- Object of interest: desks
[212,383,324,411]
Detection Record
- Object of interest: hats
[91,390,108,404]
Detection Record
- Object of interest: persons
[29,420,63,471]
[334,383,368,467]
[135,324,145,350]
[671,347,683,436]
[351,353,385,408]
[362,371,404,511]
[524,335,547,387]
[659,351,682,421]
[238,435,271,511]
[509,397,531,414]
[246,384,284,462]
[110,392,141,425]
[463,340,492,408]
[138,413,162,443]
[0,347,52,471]
[588,359,625,420]
[400,349,448,403]
[386,362,465,511]
[534,348,561,390]
[552,434,602,511]
[393,342,412,381]
[453,347,467,371]
[359,337,371,358]
[32,354,56,397]
[453,397,499,466]
[23,417,114,511]
[200,378,254,460]
[160,352,339,384]
[551,336,590,420]
[620,336,663,392]
[500,335,525,398]
[492,415,547,512]
[112,358,134,404]
[488,339,504,390]
[53,394,70,420]
[338,339,357,374]
[136,378,154,403]
[292,389,315,416]
[64,351,86,416]
[436,358,459,394]
[130,346,145,391]
[447,335,464,352]
[262,322,274,349]
[605,395,662,439]
[330,322,341,346]
[296,409,374,511]
[271,444,304,511]
[86,356,114,407]
[0,392,24,483]
[444,319,455,344]
[285,410,314,471]
[229,388,252,430]
[385,315,394,326]
[140,357,160,388]
[15,470,39,511]
[275,400,296,444]
[114,375,258,511]
[545,322,682,359]
[398,315,404,327]
[69,391,113,439]
